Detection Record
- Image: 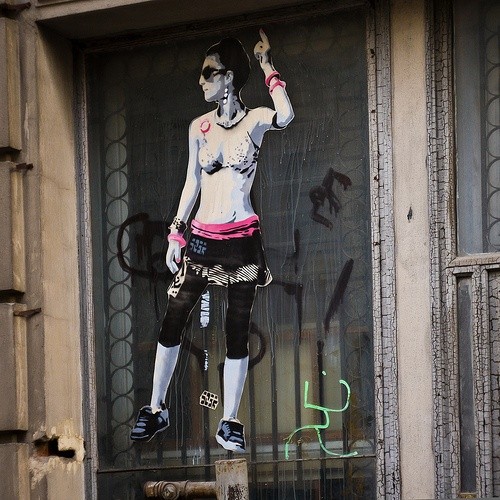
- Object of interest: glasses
[202,65,228,81]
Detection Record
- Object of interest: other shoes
[130,402,170,444]
[214,417,247,454]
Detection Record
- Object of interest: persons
[125,27,297,454]
